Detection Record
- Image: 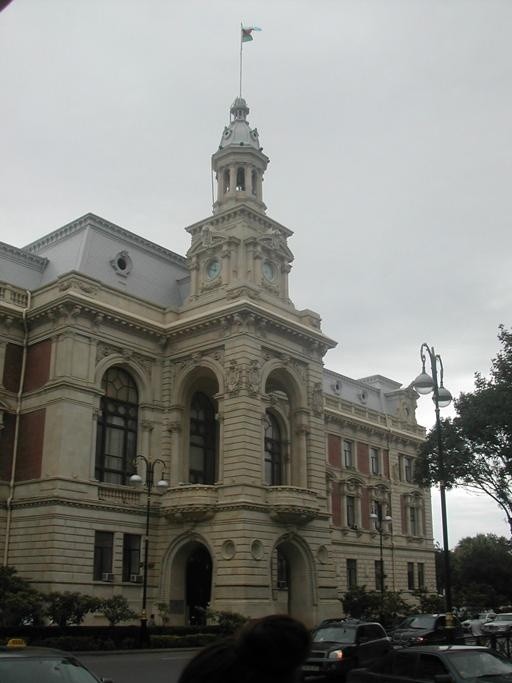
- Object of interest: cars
[0,638,113,682]
[301,606,511,683]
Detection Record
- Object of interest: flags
[241,25,263,42]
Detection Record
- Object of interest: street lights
[130,455,169,648]
[368,514,393,606]
[414,343,456,645]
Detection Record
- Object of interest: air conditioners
[102,572,114,581]
[130,574,143,583]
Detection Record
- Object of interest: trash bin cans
[451,607,457,615]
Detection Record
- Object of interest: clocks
[205,258,221,279]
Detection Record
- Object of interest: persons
[468,613,485,646]
[175,616,314,680]
